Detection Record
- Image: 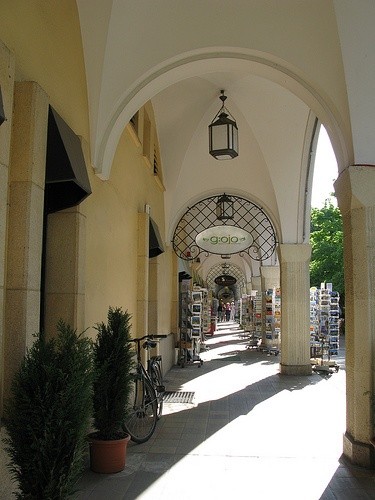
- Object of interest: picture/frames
[190,327,201,338]
[191,303,203,314]
[192,291,203,302]
[190,316,201,326]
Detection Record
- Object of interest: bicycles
[122,333,167,444]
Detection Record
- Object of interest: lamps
[216,193,234,222]
[222,263,229,274]
[208,90,239,160]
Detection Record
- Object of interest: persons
[217,299,235,322]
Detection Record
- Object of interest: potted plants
[85,305,141,474]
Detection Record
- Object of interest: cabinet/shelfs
[234,287,340,377]
[179,271,219,368]
